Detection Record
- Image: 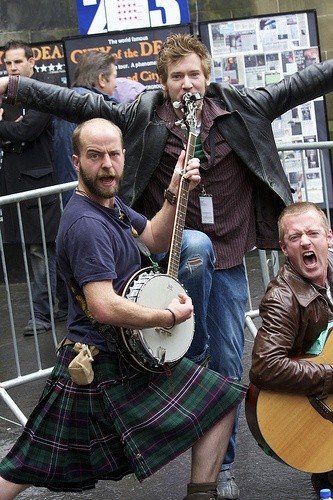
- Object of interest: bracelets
[164,309,175,330]
[7,75,17,106]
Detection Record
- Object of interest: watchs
[164,189,177,204]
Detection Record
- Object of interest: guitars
[244,326,333,474]
[110,92,205,373]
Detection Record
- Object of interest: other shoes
[217,469,238,500]
[52,312,67,322]
[24,319,45,334]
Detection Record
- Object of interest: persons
[50,51,118,208]
[250,201,333,497]
[0,118,249,500]
[0,33,333,500]
[0,40,68,336]
[228,58,236,70]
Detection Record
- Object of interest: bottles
[319,488,333,500]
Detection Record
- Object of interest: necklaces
[75,187,124,219]
